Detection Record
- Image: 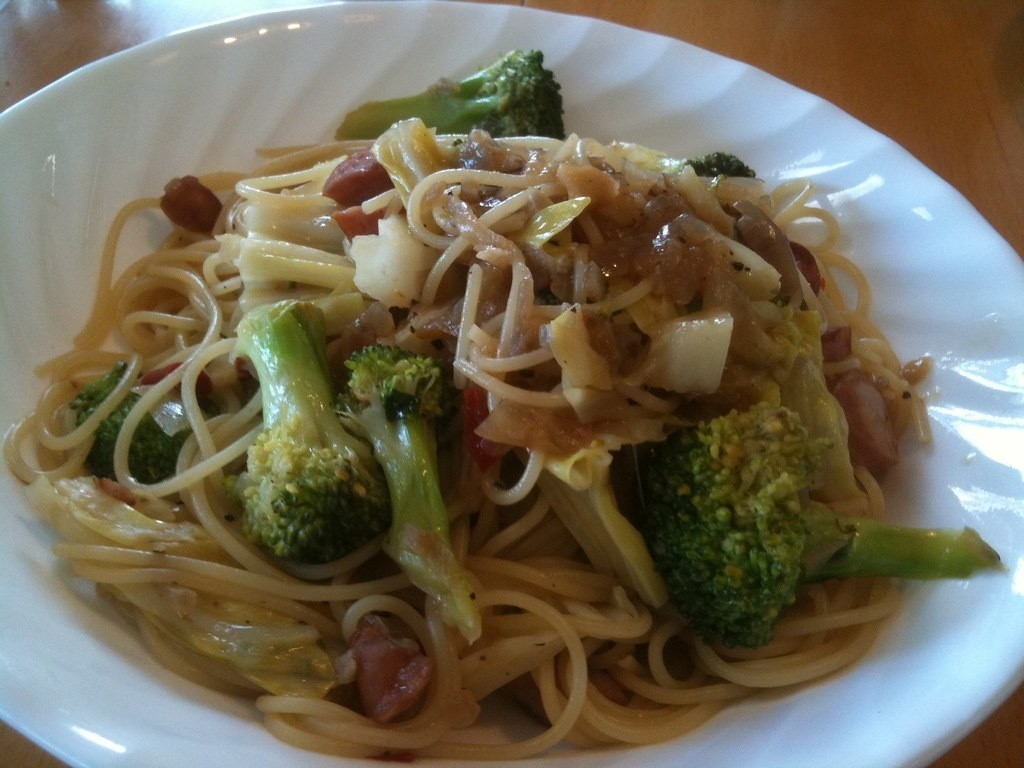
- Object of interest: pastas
[2,130,934,761]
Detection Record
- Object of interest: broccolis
[67,49,1000,651]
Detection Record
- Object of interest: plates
[0,0,1024,768]
